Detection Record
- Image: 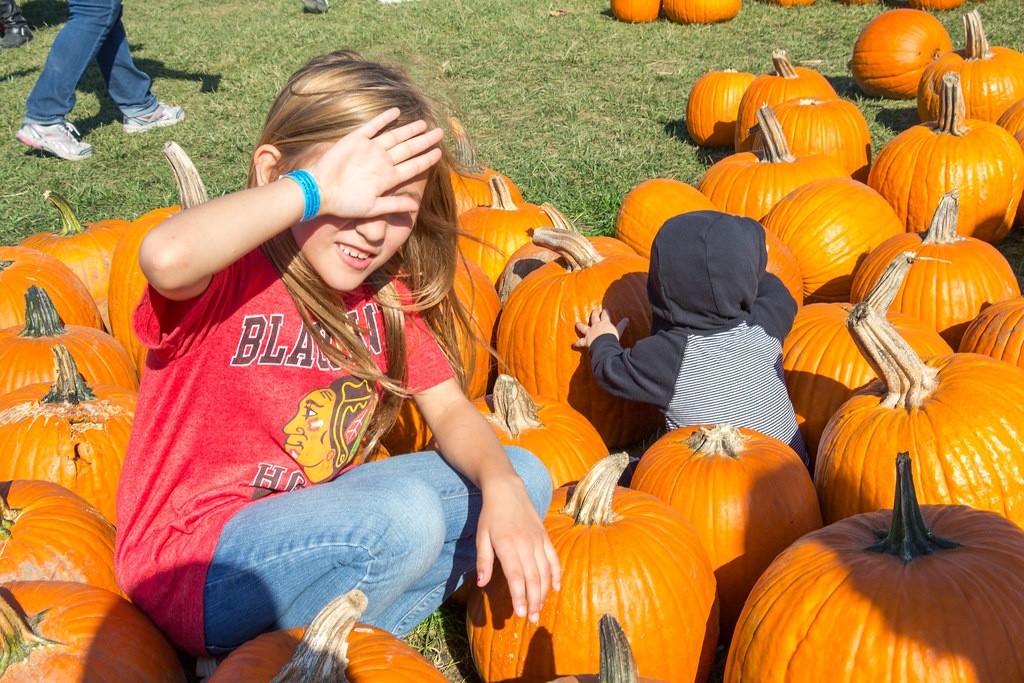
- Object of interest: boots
[0,0,34,48]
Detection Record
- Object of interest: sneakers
[122,102,185,133]
[15,124,93,161]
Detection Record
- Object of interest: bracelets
[278,168,320,224]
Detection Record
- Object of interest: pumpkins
[0,0,1024,683]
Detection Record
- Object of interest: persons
[117,52,560,664]
[13,0,186,161]
[570,209,811,481]
